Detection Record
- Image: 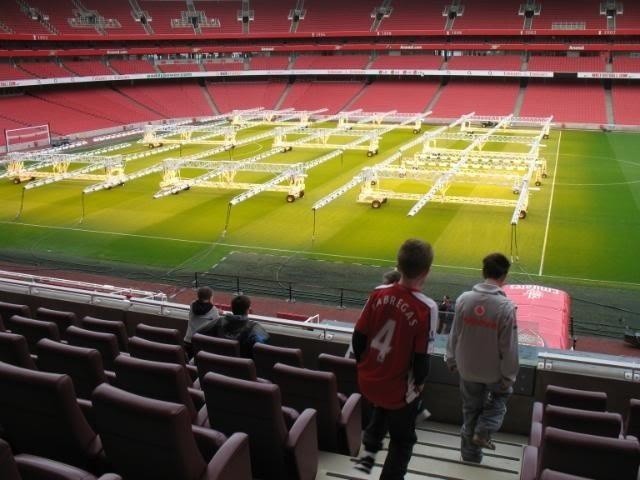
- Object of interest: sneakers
[472,432,495,450]
[352,455,375,473]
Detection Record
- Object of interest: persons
[443,252,519,465]
[436,295,450,335]
[443,303,456,335]
[382,270,432,425]
[351,237,439,480]
[191,295,272,362]
[182,285,220,356]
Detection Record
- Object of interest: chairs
[520,384,640,479]
